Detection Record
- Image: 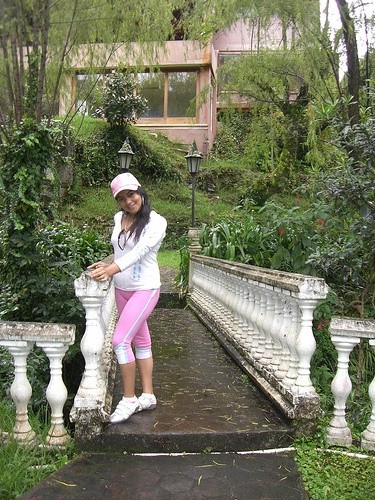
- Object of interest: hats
[110,173,141,199]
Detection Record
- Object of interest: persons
[87,171,168,424]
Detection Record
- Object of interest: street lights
[185,141,204,227]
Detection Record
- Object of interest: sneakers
[108,395,139,424]
[138,393,157,410]
